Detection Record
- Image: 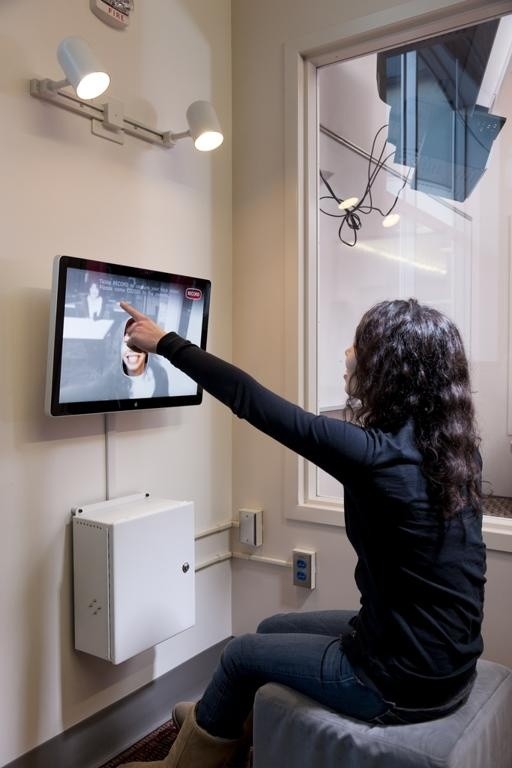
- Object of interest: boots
[117,701,236,767]
[172,701,195,729]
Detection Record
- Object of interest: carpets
[97,714,253,768]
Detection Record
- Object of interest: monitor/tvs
[43,254,212,420]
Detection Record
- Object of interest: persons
[76,281,106,320]
[113,294,484,768]
[83,316,169,401]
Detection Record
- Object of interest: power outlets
[292,548,316,590]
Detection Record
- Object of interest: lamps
[316,126,417,249]
[28,34,226,154]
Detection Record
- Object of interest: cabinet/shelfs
[72,492,198,668]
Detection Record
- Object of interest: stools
[251,654,511,768]
